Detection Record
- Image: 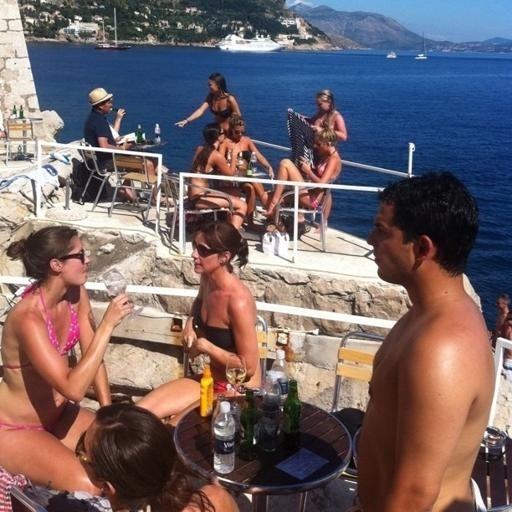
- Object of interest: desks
[6,117,45,159]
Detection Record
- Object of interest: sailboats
[94,7,132,50]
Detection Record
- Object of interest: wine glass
[226,355,247,406]
[102,268,144,321]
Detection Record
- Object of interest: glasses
[235,130,245,134]
[193,241,223,257]
[59,249,84,264]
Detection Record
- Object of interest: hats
[88,88,113,106]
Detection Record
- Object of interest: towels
[286,111,315,181]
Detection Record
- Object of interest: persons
[84,87,173,208]
[351,172,496,511]
[174,74,243,141]
[488,294,511,350]
[498,311,512,358]
[73,401,242,512]
[189,89,347,239]
[0,224,140,495]
[129,220,264,419]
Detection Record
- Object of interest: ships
[217,33,285,54]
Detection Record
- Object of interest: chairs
[76,136,330,253]
[4,114,41,163]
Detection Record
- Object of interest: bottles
[111,108,128,120]
[249,152,258,173]
[214,402,235,473]
[135,122,145,143]
[227,152,232,167]
[13,105,16,113]
[283,380,302,432]
[200,365,214,417]
[20,105,23,118]
[260,372,281,452]
[239,389,258,459]
[154,123,161,143]
[237,151,243,163]
[271,351,288,411]
[211,393,225,458]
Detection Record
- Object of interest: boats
[415,54,427,60]
[387,50,398,59]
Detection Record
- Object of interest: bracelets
[183,117,190,125]
